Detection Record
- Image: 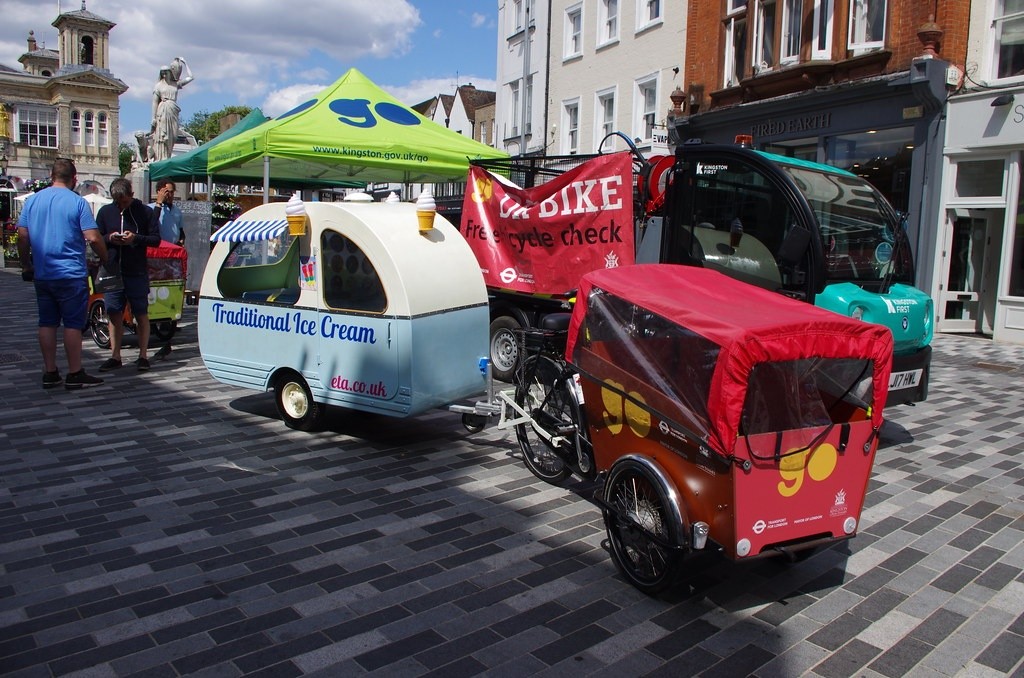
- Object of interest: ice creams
[385,191,400,202]
[416,188,436,231]
[285,193,307,236]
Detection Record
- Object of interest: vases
[5,260,20,267]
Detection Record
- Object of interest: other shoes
[159,322,181,331]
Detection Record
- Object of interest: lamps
[991,94,1015,105]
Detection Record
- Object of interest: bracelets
[156,201,163,206]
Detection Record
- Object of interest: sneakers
[99,358,122,371]
[43,367,63,389]
[137,358,149,369]
[65,369,105,390]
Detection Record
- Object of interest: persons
[96,178,161,372]
[146,179,185,332]
[16,159,105,389]
[146,57,194,159]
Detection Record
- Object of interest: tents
[150,107,367,209]
[207,65,511,265]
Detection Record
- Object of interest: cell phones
[114,234,128,238]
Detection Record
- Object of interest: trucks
[467,134,934,409]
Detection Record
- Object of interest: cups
[286,215,307,236]
[417,209,436,230]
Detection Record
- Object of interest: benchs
[587,336,707,413]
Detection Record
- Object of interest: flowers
[3,233,20,260]
[211,188,243,235]
